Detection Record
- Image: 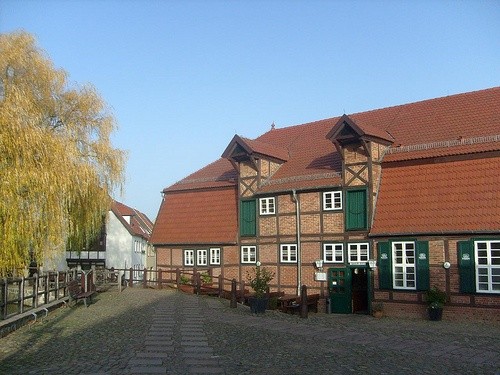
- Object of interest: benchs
[192,288,320,319]
[65,280,96,308]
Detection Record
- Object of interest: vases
[249,298,269,315]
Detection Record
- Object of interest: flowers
[246,260,277,299]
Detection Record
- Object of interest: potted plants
[373,302,384,317]
[422,285,448,321]
[177,274,192,293]
[202,271,214,290]
